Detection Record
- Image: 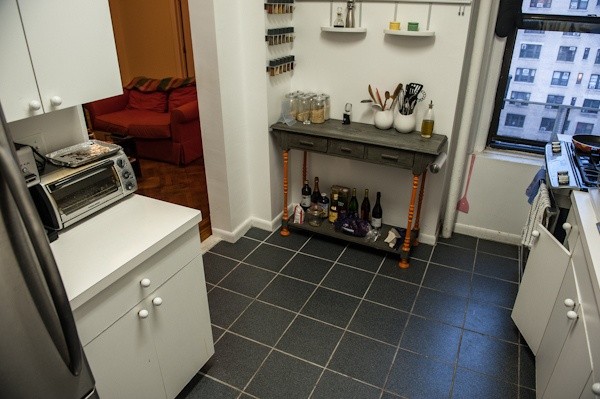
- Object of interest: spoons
[382,90,390,110]
[371,104,382,111]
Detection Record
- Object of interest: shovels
[405,83,423,106]
[456,153,476,215]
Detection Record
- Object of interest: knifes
[368,84,377,104]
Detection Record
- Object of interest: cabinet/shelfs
[535,258,600,399]
[1,1,124,124]
[83,75,202,167]
[50,194,215,399]
[510,203,580,357]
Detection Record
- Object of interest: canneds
[296,96,312,121]
[311,95,325,123]
[287,93,298,120]
[306,92,317,97]
[294,89,304,95]
[319,93,330,121]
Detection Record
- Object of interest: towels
[525,168,545,204]
[520,183,552,252]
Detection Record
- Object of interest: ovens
[520,173,570,281]
[28,150,139,231]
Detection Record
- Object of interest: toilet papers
[430,153,447,173]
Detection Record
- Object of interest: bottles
[269,55,294,77]
[394,110,417,134]
[349,188,359,218]
[302,180,311,211]
[311,177,321,205]
[284,90,330,123]
[361,189,370,222]
[268,26,294,47]
[345,1,355,28]
[333,7,344,27]
[374,110,393,130]
[267,0,293,15]
[420,100,435,138]
[372,192,382,229]
[317,193,329,218]
[329,194,339,223]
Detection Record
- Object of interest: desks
[269,114,448,269]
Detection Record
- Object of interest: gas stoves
[545,141,600,191]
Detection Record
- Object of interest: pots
[572,133,600,155]
